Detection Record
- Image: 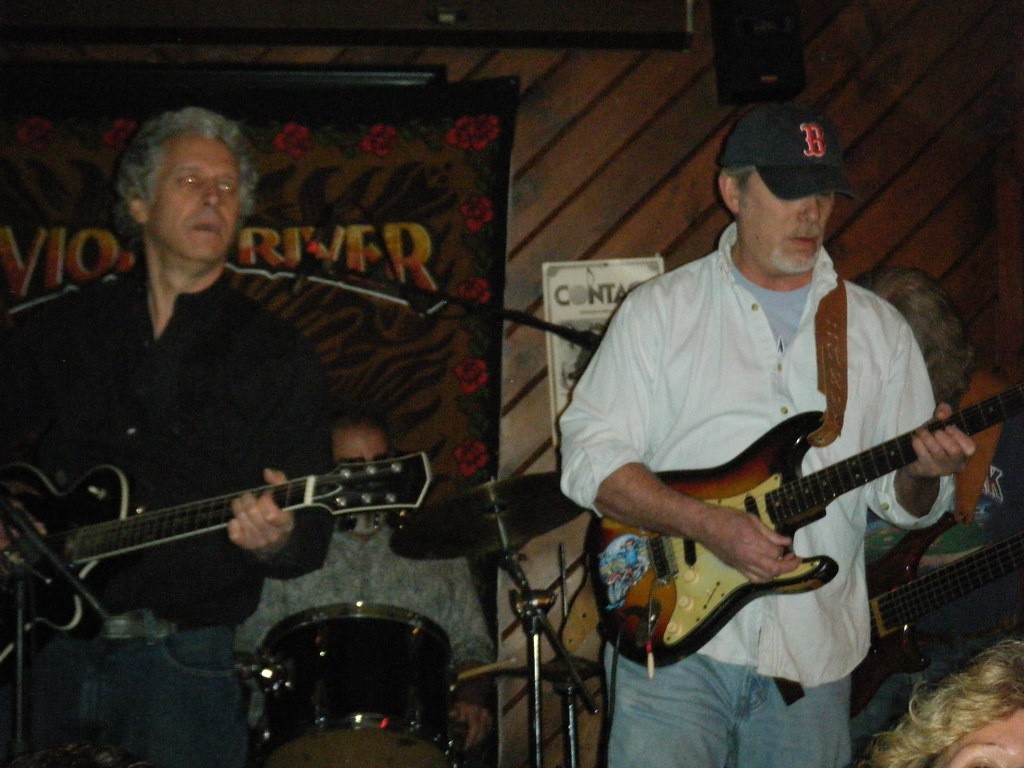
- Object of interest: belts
[95,611,180,640]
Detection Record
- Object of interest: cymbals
[390,472,582,562]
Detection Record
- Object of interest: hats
[718,99,857,199]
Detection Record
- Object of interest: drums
[238,597,464,768]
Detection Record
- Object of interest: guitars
[2,456,441,668]
[837,507,1023,679]
[585,385,1023,674]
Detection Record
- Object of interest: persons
[851,266,1023,756]
[557,110,975,767]
[231,405,495,750]
[859,639,1024,767]
[0,105,336,768]
